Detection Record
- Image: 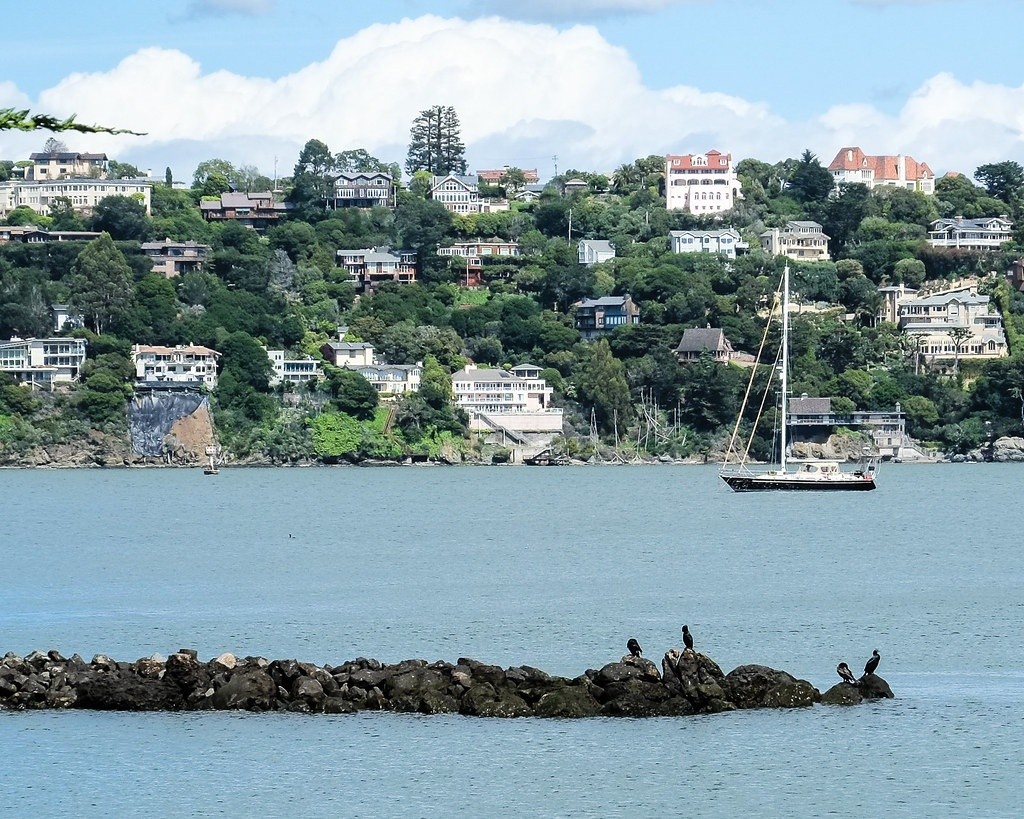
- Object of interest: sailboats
[716,258,875,492]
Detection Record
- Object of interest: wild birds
[836,661,857,683]
[862,649,882,677]
[626,638,644,658]
[680,625,694,650]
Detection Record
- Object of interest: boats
[204,466,220,474]
